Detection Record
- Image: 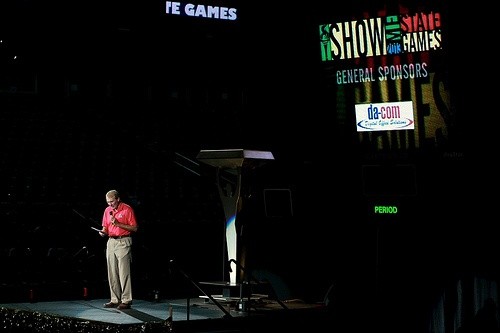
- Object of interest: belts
[109,235,131,239]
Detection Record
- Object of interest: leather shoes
[103,301,118,308]
[116,303,130,310]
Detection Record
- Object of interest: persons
[99,190,137,309]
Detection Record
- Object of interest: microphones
[109,211,114,226]
